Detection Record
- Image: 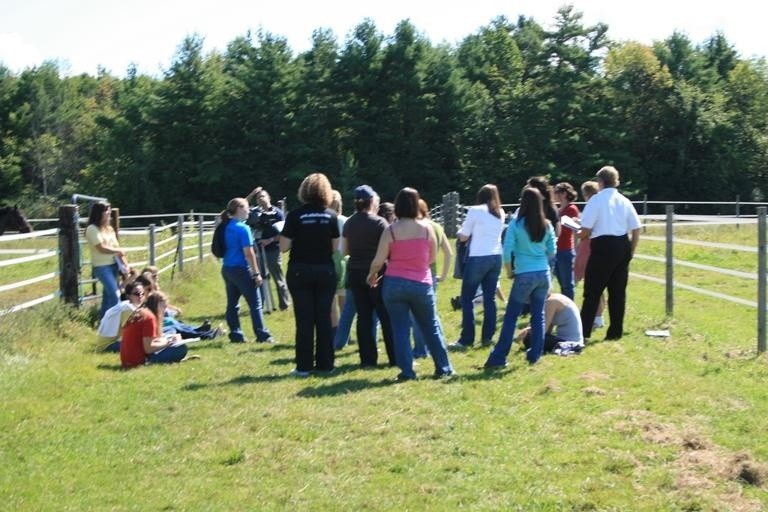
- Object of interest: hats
[352,184,374,200]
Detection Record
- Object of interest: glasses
[131,291,145,297]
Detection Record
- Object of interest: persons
[120,294,188,369]
[378,198,451,359]
[277,172,341,376]
[327,190,355,350]
[85,200,130,324]
[573,165,643,341]
[444,183,505,351]
[340,181,398,368]
[367,185,455,383]
[480,186,558,370]
[514,176,607,355]
[94,266,222,354]
[221,185,289,344]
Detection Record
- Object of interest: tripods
[251,229,276,315]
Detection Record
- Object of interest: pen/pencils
[371,275,383,287]
[255,284,259,290]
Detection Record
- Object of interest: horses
[0,204,34,236]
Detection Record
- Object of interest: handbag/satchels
[453,234,472,279]
[211,217,233,257]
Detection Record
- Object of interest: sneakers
[288,368,310,377]
[310,366,336,376]
[208,322,226,340]
